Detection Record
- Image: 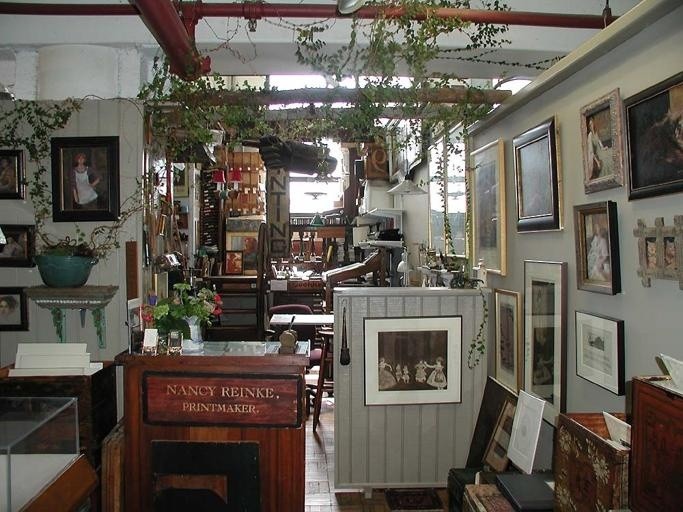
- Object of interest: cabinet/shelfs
[0,395,100,511]
[350,207,405,283]
[629,377,683,512]
[0,359,117,512]
[226,151,267,224]
[332,286,488,500]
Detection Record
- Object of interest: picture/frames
[470,139,507,276]
[494,287,523,395]
[0,135,121,332]
[363,315,463,407]
[573,200,621,296]
[523,260,568,430]
[114,340,311,512]
[290,224,346,254]
[385,100,427,184]
[481,400,516,474]
[269,314,334,432]
[202,275,267,342]
[222,250,244,276]
[512,115,561,232]
[575,310,625,396]
[623,74,679,202]
[633,215,681,288]
[384,117,427,185]
[506,391,545,475]
[579,88,624,194]
[225,230,259,276]
[446,120,472,258]
[428,133,446,259]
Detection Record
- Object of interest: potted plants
[222,250,244,276]
[384,117,427,185]
[0,135,121,332]
[385,100,427,184]
[363,315,463,407]
[225,230,259,276]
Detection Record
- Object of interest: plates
[0,395,100,511]
[350,207,405,283]
[332,286,488,500]
[0,359,117,512]
[629,377,683,512]
[226,151,267,224]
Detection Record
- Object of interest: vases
[181,316,203,342]
[131,282,224,335]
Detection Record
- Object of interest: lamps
[268,304,323,420]
[387,119,428,195]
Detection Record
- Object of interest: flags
[181,316,203,342]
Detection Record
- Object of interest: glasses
[387,119,428,195]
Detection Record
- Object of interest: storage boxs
[552,411,631,512]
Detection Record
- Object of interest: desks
[290,224,346,254]
[114,340,311,512]
[269,314,334,432]
[202,275,267,342]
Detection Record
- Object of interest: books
[552,411,631,512]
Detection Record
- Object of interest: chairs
[268,304,323,420]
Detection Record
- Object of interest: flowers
[131,282,224,335]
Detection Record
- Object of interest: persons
[68,153,100,210]
[0,237,24,258]
[587,117,610,180]
[0,158,15,192]
[378,357,447,390]
[587,224,609,281]
[0,296,17,317]
